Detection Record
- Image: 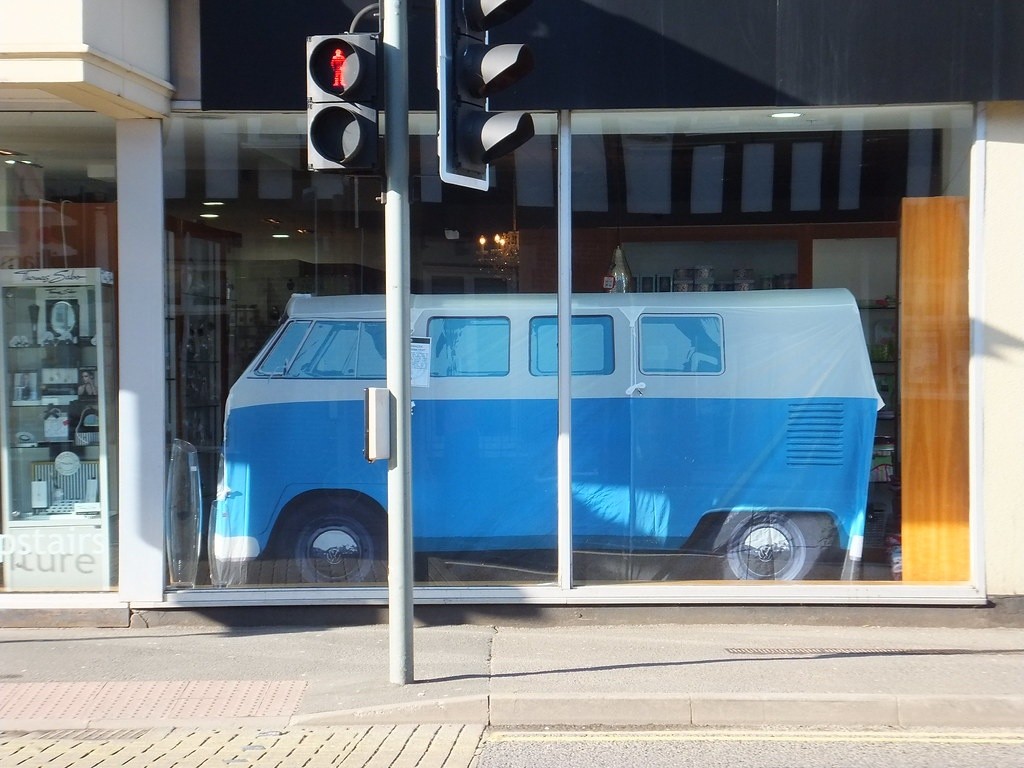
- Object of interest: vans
[209,289,877,588]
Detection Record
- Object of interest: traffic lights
[306,32,382,175]
[435,0,535,191]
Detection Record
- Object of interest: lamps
[605,134,636,294]
[475,231,520,282]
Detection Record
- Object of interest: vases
[205,499,235,587]
[167,439,204,589]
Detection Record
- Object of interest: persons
[18,374,32,401]
[78,372,98,395]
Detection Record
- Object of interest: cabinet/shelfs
[0,268,115,593]
[160,215,245,564]
[798,221,902,561]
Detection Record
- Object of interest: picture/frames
[35,287,89,344]
[11,370,42,401]
[78,366,98,399]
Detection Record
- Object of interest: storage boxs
[43,412,68,438]
[863,502,889,546]
